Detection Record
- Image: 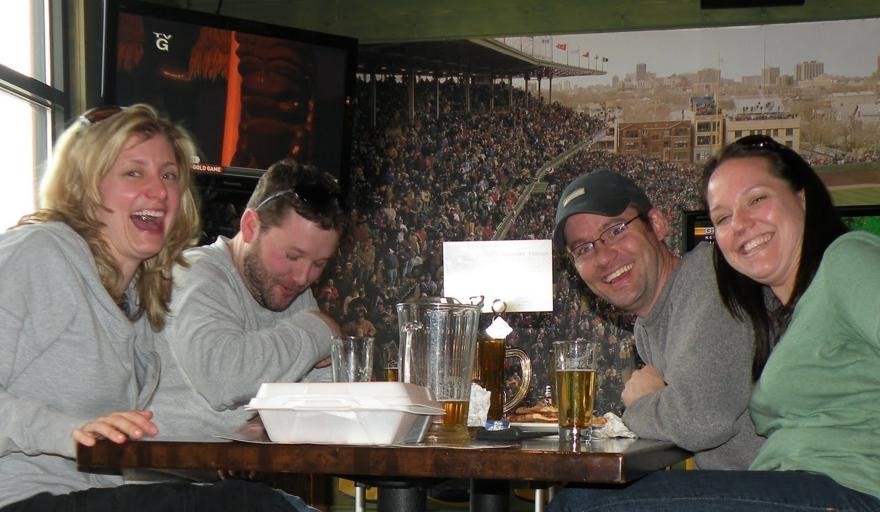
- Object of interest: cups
[330,336,373,383]
[395,302,480,447]
[384,360,397,382]
[440,339,533,433]
[552,339,598,443]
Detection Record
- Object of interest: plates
[505,417,608,434]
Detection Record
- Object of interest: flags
[540,38,609,63]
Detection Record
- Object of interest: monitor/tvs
[102,0,359,195]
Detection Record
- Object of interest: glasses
[734,135,771,149]
[569,213,641,259]
[255,183,350,213]
[79,103,122,125]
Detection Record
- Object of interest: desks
[76,434,696,512]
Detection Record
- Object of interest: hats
[552,171,653,254]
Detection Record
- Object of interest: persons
[541,135,880,512]
[554,170,768,472]
[0,100,327,512]
[136,158,342,442]
